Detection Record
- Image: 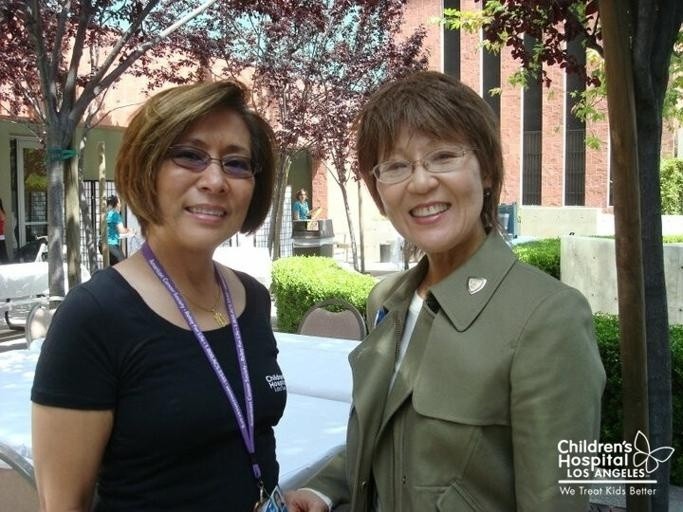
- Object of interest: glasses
[168,144,266,180]
[367,146,475,184]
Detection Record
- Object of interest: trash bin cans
[291,219,337,257]
[498,201,519,239]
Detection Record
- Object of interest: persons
[24,77,287,511]
[292,187,310,221]
[285,68,607,510]
[97,193,132,262]
[0,198,10,264]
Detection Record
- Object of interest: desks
[1,332,362,493]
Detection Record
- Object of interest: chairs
[0,442,38,512]
[297,301,367,341]
[25,303,52,348]
